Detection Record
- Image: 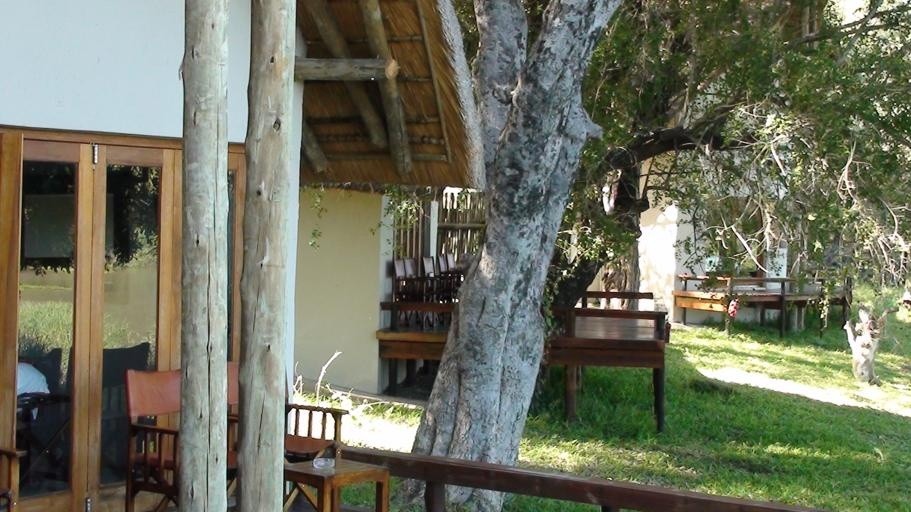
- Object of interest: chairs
[65,342,149,478]
[124,367,235,512]
[393,253,462,329]
[226,362,350,512]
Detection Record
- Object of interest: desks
[284,457,392,512]
